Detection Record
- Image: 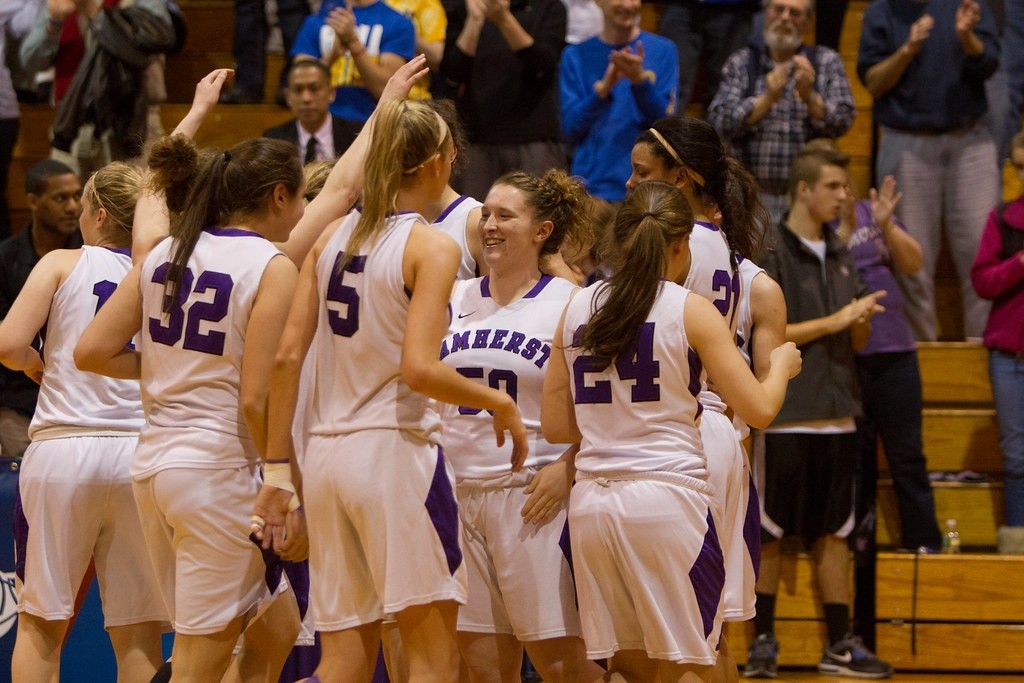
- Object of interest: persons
[557,0,733,203]
[382,0,448,99]
[624,115,787,683]
[708,0,853,261]
[251,97,530,683]
[976,0,1024,183]
[294,0,416,120]
[72,52,429,683]
[740,148,893,679]
[806,140,943,553]
[438,0,567,203]
[539,180,802,683]
[856,0,1001,341]
[0,159,163,683]
[0,0,319,461]
[970,130,1024,555]
[428,170,613,683]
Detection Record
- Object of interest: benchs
[719,342,1024,673]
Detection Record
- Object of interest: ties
[303,137,318,164]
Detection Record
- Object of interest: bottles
[941,519,961,554]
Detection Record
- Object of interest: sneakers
[741,641,778,678]
[818,637,891,679]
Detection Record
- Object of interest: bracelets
[351,45,368,56]
